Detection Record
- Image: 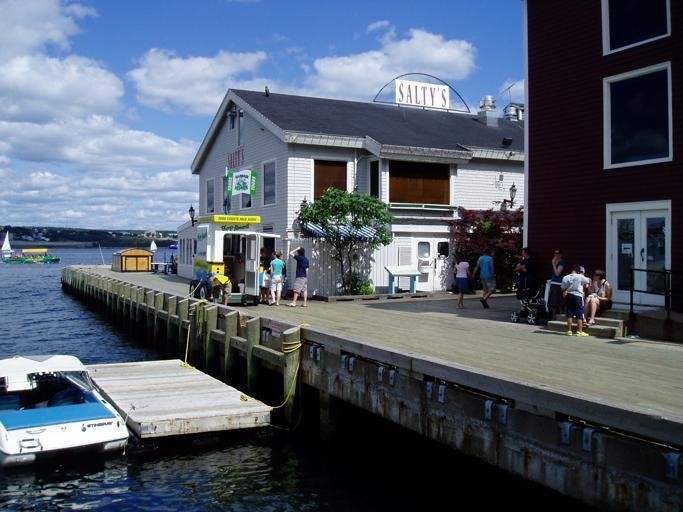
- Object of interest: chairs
[47,388,81,407]
[0,394,21,410]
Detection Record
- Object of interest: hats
[205,273,212,279]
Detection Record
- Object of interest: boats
[0,357,133,468]
[2,247,61,265]
[169,240,178,249]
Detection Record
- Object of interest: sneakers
[574,331,590,338]
[260,300,308,308]
[480,298,490,310]
[457,305,466,310]
[565,331,573,337]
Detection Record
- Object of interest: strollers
[509,280,548,325]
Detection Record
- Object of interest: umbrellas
[167,241,178,250]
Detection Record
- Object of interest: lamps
[503,182,516,210]
[189,204,197,226]
[502,137,513,150]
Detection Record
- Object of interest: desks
[149,262,174,276]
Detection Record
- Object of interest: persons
[471,246,496,309]
[259,266,271,305]
[267,250,284,306]
[559,263,590,337]
[580,268,613,327]
[14,251,47,259]
[512,247,534,299]
[453,253,472,309]
[561,266,592,326]
[285,245,309,307]
[206,274,232,306]
[546,247,567,320]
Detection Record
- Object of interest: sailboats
[1,230,15,254]
[148,240,158,252]
